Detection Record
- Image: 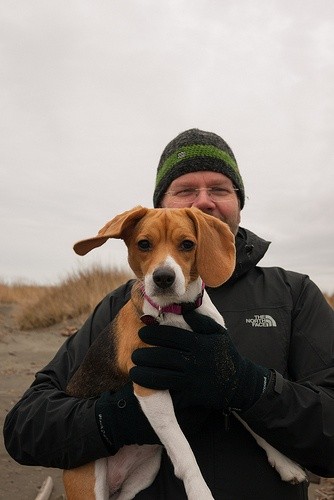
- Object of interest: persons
[2,129,333,500]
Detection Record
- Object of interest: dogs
[61,205,308,500]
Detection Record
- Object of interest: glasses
[164,183,240,203]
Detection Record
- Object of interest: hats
[152,130,245,209]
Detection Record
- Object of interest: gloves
[129,305,269,414]
[96,386,201,449]
